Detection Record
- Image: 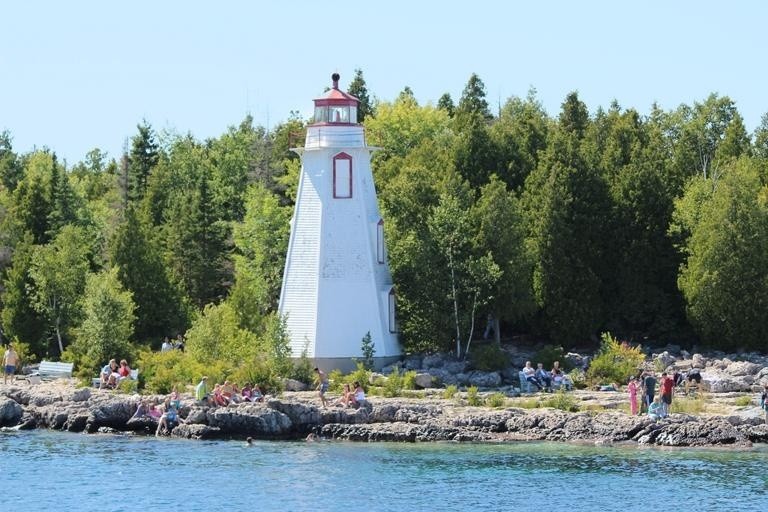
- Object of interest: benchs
[92,369,138,389]
[518,371,571,393]
[39,361,74,381]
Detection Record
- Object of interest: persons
[161,337,175,352]
[174,334,185,352]
[523,360,547,392]
[114,359,132,391]
[760,386,768,424]
[132,392,187,439]
[582,352,594,373]
[98,359,119,389]
[312,367,330,408]
[106,372,122,390]
[194,376,264,406]
[328,383,351,409]
[628,370,673,422]
[550,360,572,390]
[346,380,365,407]
[535,362,552,390]
[1,344,20,385]
[687,363,703,395]
